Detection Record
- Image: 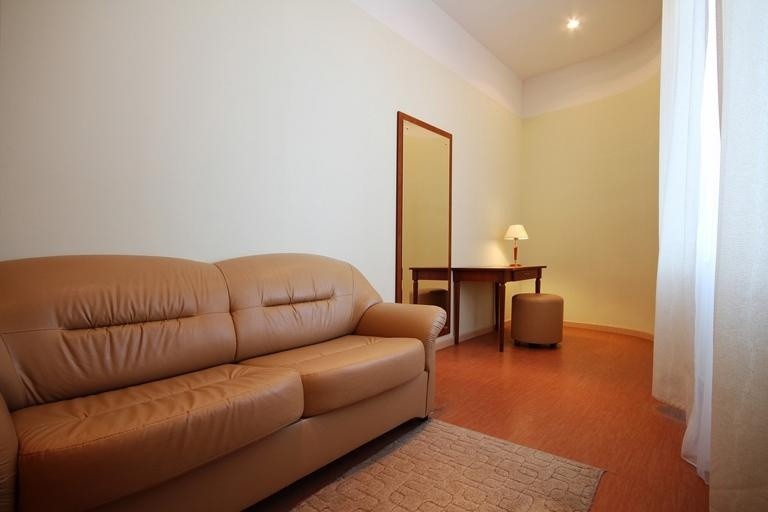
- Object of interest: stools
[511,293,565,350]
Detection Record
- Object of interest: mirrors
[395,111,453,338]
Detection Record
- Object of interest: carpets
[281,416,606,511]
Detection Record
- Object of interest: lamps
[504,224,528,267]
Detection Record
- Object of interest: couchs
[2,251,447,512]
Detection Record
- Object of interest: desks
[452,266,546,352]
[408,267,448,305]
